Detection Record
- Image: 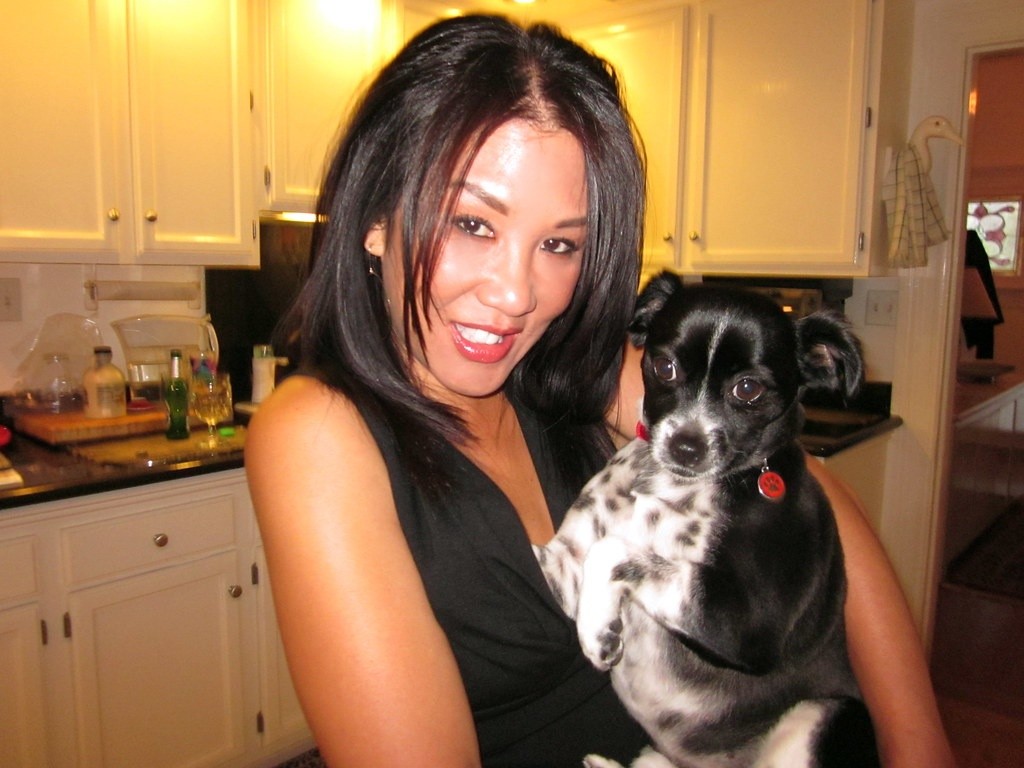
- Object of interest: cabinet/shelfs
[0,429,322,768]
[0,1,916,284]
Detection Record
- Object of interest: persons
[243,15,957,768]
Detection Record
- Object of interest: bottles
[85,346,126,418]
[165,349,190,439]
[251,345,276,403]
[44,354,72,413]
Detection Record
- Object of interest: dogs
[528,269,881,768]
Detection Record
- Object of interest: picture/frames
[965,195,1024,277]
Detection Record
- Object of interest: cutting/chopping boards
[7,403,233,443]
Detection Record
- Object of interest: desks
[944,364,1024,566]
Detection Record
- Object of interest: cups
[128,361,170,403]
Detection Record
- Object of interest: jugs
[111,313,219,404]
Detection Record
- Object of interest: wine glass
[189,372,233,450]
